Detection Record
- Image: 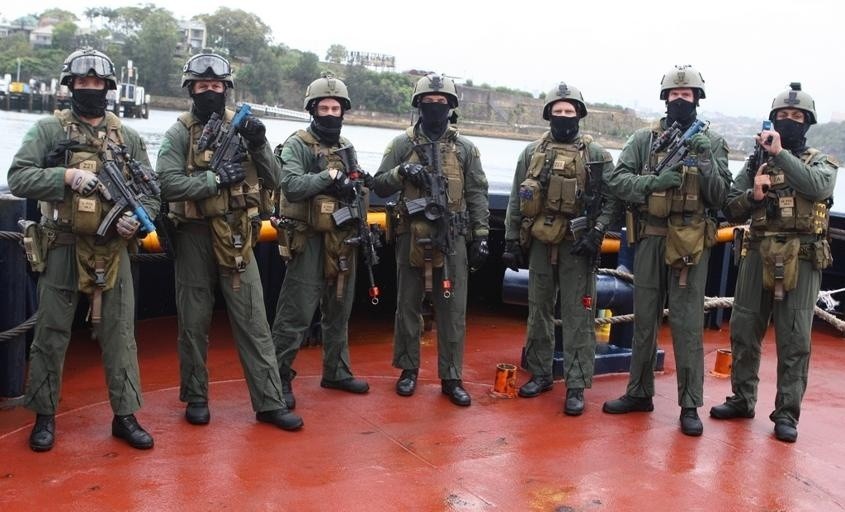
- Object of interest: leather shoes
[604,393,653,414]
[112,414,153,449]
[186,402,210,424]
[565,388,585,415]
[30,413,54,451]
[256,378,302,430]
[396,368,418,395]
[518,374,553,396]
[775,424,797,442]
[710,402,755,418]
[680,406,703,436]
[441,379,470,406]
[321,376,369,392]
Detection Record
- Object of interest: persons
[377,71,490,408]
[157,48,303,432]
[271,76,374,411]
[7,47,162,452]
[711,82,840,443]
[504,82,617,415]
[603,63,734,436]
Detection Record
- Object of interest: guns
[405,142,467,298]
[649,120,710,175]
[331,146,383,305]
[746,121,779,230]
[569,161,601,312]
[96,153,161,236]
[198,104,251,173]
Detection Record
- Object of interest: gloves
[646,163,683,192]
[467,237,489,273]
[398,162,432,192]
[116,210,141,239]
[333,169,356,203]
[501,239,524,272]
[685,133,714,161]
[359,169,375,191]
[215,152,247,188]
[235,115,266,146]
[72,169,112,201]
[568,228,604,257]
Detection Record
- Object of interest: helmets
[768,83,817,124]
[303,75,352,112]
[660,65,706,100]
[410,73,458,108]
[59,46,118,90]
[180,54,234,89]
[542,82,588,121]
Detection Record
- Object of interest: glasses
[183,55,233,78]
[62,55,116,78]
